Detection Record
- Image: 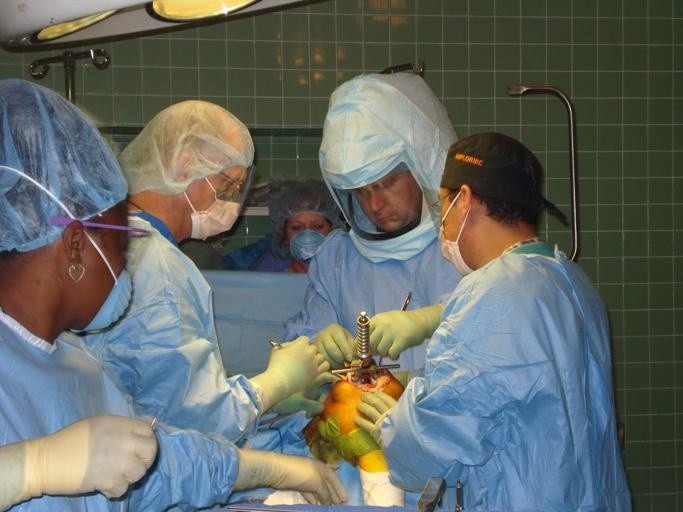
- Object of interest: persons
[276,71,468,415]
[1,79,346,511]
[70,100,343,443]
[356,133,633,512]
[223,179,352,274]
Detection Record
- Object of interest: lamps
[0,0,324,53]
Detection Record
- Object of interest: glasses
[429,192,454,215]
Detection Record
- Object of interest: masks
[438,190,475,277]
[285,228,325,266]
[186,179,244,239]
[67,227,134,332]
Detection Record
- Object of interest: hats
[441,132,543,208]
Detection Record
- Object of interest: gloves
[233,448,348,506]
[0,414,158,512]
[356,303,446,360]
[248,323,354,417]
[354,390,397,449]
